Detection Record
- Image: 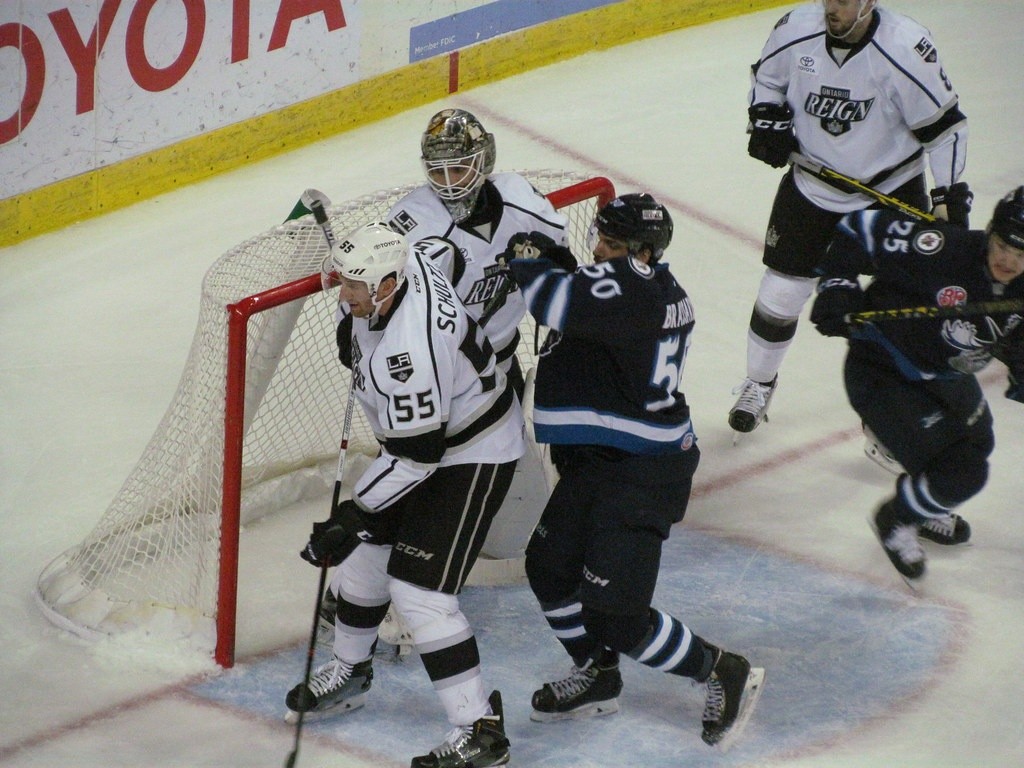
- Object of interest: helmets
[421,109,497,200]
[593,193,674,268]
[328,221,409,307]
[990,186,1024,250]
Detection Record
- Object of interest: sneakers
[873,475,928,590]
[410,688,511,768]
[857,418,911,477]
[918,510,972,549]
[529,653,624,723]
[286,653,373,726]
[727,373,779,446]
[700,650,766,757]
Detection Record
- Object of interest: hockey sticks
[284,374,357,768]
[834,300,1024,326]
[310,199,336,251]
[788,150,937,222]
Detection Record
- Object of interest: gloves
[923,182,974,243]
[506,230,576,275]
[336,312,354,371]
[746,102,801,169]
[809,291,862,339]
[299,500,373,568]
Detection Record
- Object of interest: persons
[811,184,1024,593]
[318,108,571,663]
[284,221,527,768]
[727,0,969,447]
[503,192,765,755]
